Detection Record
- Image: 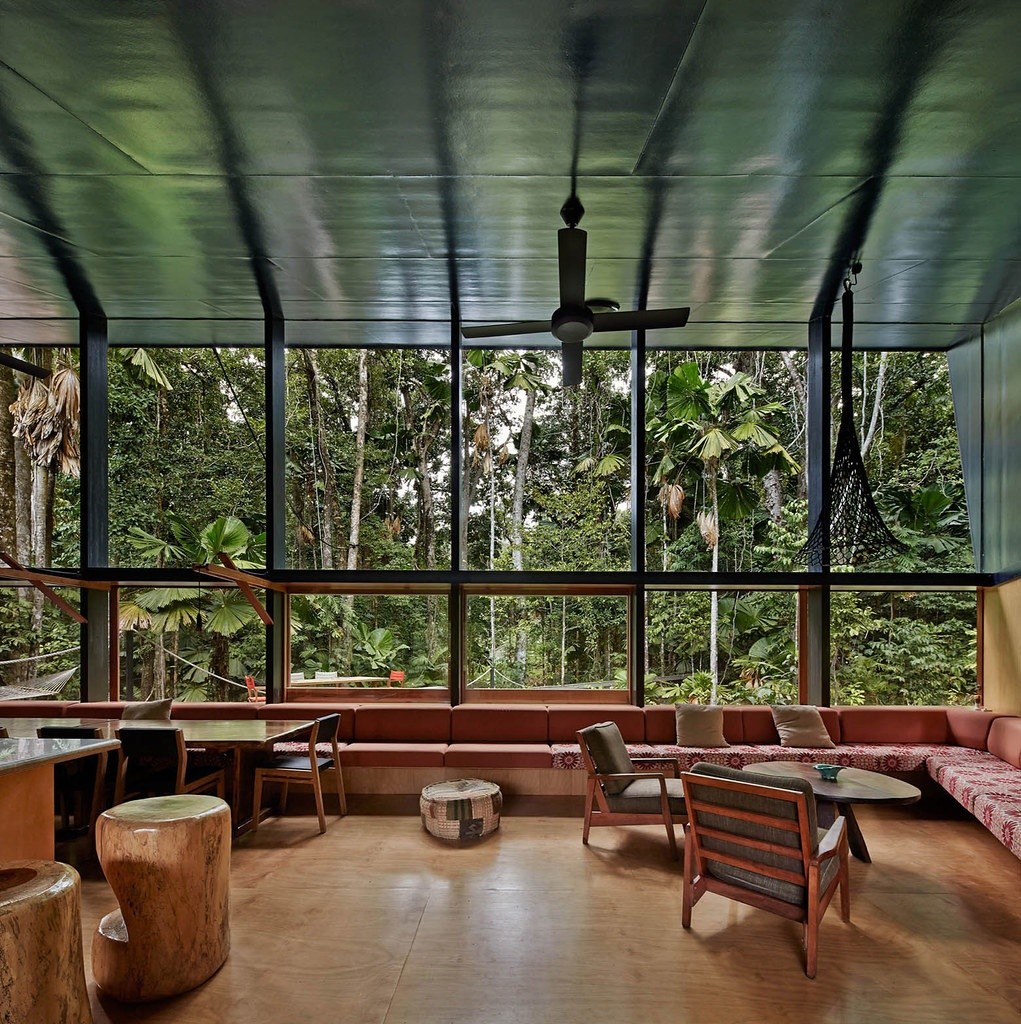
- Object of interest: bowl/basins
[813,763,845,780]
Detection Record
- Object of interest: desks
[0,738,123,861]
[0,717,317,836]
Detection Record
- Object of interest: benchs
[29,702,1020,864]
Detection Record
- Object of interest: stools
[0,858,91,1024]
[420,777,505,839]
[92,793,232,1000]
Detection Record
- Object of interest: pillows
[770,705,837,751]
[674,702,730,747]
[121,699,173,721]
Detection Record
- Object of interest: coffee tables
[742,761,921,863]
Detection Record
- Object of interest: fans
[460,197,690,388]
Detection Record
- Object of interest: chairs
[114,726,226,807]
[254,714,347,835]
[37,726,108,846]
[682,762,851,978]
[577,721,692,859]
